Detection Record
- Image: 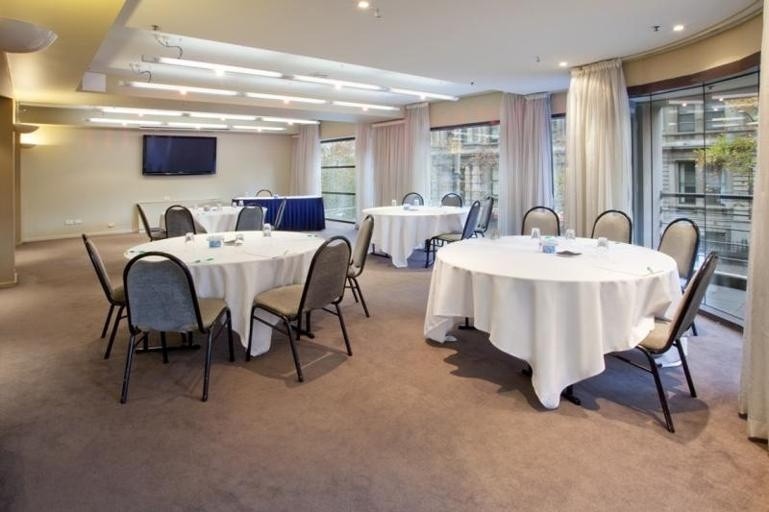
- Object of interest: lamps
[1,19,56,53]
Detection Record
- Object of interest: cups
[565,228,576,244]
[184,231,195,243]
[263,223,272,238]
[595,237,608,255]
[530,228,540,240]
[414,200,420,206]
[236,233,244,246]
[392,199,397,207]
[193,200,244,211]
[273,192,278,198]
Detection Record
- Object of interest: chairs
[400,191,422,207]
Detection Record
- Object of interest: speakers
[82,71,106,93]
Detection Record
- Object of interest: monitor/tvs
[143,135,217,175]
